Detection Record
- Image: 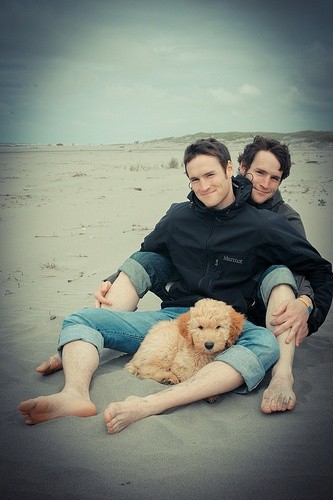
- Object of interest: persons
[37,135,314,414]
[18,138,333,434]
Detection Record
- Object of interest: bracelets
[298,297,312,315]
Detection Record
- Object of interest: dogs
[124,298,246,404]
